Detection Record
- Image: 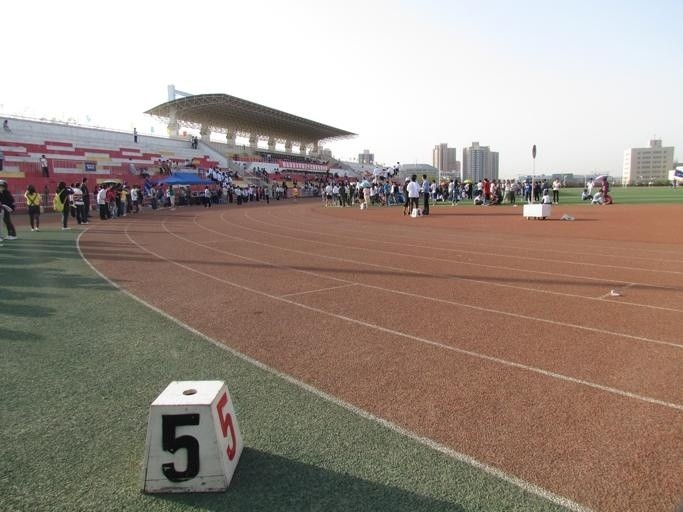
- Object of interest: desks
[523,204,551,220]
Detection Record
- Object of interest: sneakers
[35,227,40,231]
[4,235,17,240]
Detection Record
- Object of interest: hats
[0,180,6,185]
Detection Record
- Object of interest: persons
[93,182,143,220]
[3,119,11,133]
[58,177,91,229]
[133,127,138,144]
[473,178,561,205]
[581,178,612,205]
[24,184,43,232]
[41,155,50,177]
[402,174,473,216]
[190,136,198,149]
[206,166,301,204]
[142,159,212,209]
[303,162,401,208]
[1,180,17,239]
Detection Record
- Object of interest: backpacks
[53,188,67,211]
